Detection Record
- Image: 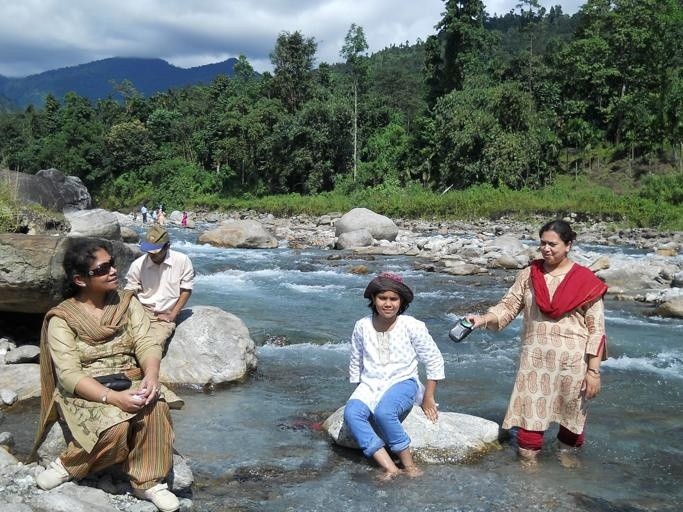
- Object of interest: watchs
[587,367,602,374]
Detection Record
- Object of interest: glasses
[82,255,115,278]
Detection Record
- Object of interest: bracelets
[479,316,488,331]
[588,372,601,379]
[101,388,112,406]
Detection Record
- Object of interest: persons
[460,220,609,472]
[132,203,189,230]
[348,272,447,485]
[123,230,196,357]
[26,235,181,512]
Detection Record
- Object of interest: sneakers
[134,482,180,512]
[35,458,73,490]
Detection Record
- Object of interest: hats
[139,227,170,254]
[364,272,413,305]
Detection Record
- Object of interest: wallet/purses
[93,372,132,391]
